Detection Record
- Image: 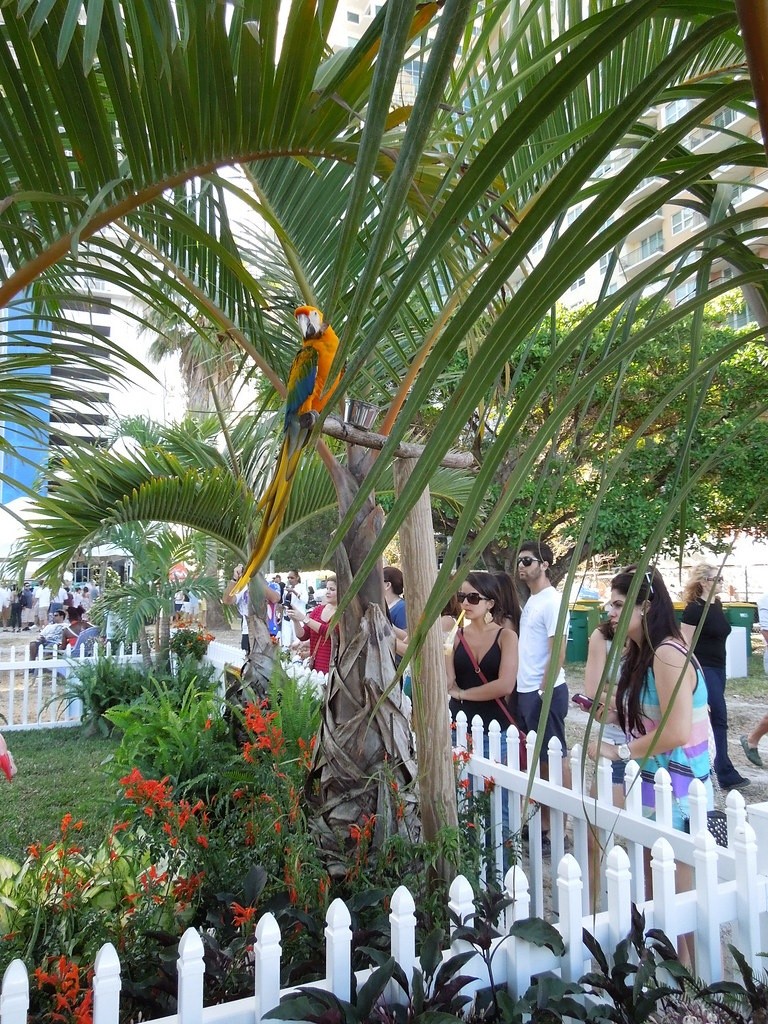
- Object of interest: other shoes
[723,778,751,791]
[17,629,21,632]
[12,629,15,632]
[22,627,30,631]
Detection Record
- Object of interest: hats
[276,575,281,578]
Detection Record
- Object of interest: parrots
[230,305,344,597]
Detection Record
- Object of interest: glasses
[287,576,294,580]
[26,586,28,587]
[54,615,58,616]
[630,568,653,593]
[517,556,540,567]
[457,592,490,605]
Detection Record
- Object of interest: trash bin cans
[721,603,756,655]
[598,611,609,623]
[316,574,327,590]
[576,600,604,637]
[566,604,588,663]
[672,603,688,622]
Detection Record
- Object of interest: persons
[444,571,519,894]
[516,541,570,859]
[270,575,286,631]
[491,571,521,716]
[579,573,717,966]
[582,565,650,998]
[739,588,768,766]
[0,580,99,674]
[381,566,408,690]
[286,575,338,673]
[281,569,307,646]
[680,562,751,791]
[174,589,185,621]
[307,586,316,606]
[389,571,464,731]
[222,562,281,650]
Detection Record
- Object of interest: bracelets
[459,689,464,704]
[230,576,237,582]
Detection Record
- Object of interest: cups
[443,631,454,656]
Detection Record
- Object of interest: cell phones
[572,694,603,712]
[285,600,293,610]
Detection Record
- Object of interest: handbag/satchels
[684,811,727,847]
[519,731,527,772]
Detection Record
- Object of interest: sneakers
[740,734,763,766]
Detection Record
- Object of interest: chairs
[33,626,100,680]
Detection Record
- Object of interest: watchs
[618,742,632,763]
[303,616,311,624]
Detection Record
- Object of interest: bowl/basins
[344,398,382,431]
